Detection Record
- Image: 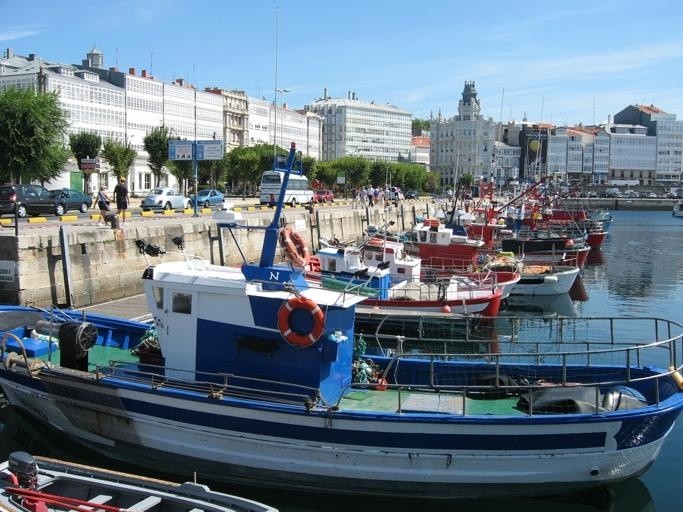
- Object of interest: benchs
[67,494,112,512]
[126,495,162,512]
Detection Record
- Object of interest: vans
[259,171,313,207]
[0,184,67,218]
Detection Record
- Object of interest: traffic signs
[80,169,100,174]
[80,164,100,168]
[80,158,99,163]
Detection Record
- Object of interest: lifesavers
[463,224,475,236]
[283,228,311,266]
[505,216,515,225]
[367,237,384,246]
[279,296,324,346]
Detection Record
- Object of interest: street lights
[275,88,289,170]
[191,84,197,217]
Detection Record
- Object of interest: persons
[112,177,130,226]
[348,184,400,210]
[93,185,115,226]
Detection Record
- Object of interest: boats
[353,321,501,361]
[672,201,682,217]
[0,451,280,512]
[0,142,682,500]
[270,171,615,332]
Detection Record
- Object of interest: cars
[138,188,192,213]
[569,187,683,198]
[187,189,225,208]
[47,187,92,214]
[354,186,474,204]
